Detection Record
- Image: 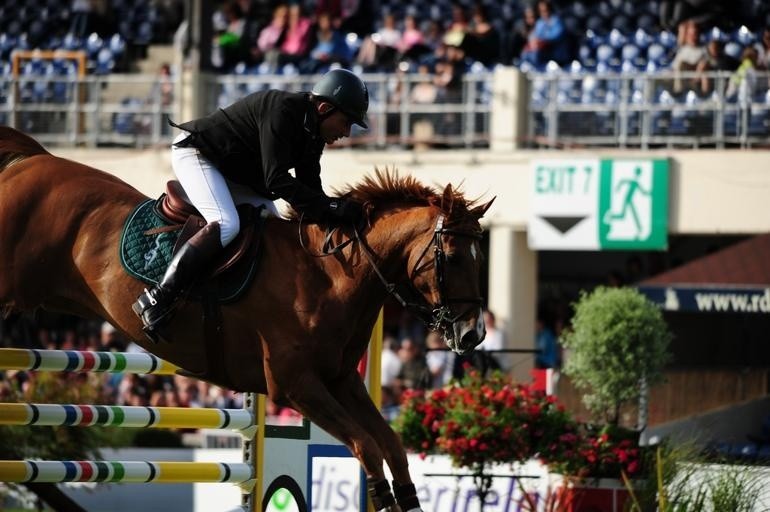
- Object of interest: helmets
[309,68,370,130]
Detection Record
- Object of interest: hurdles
[1,307,383,511]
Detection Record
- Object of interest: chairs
[0,1,770,135]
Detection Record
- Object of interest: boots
[131,221,225,344]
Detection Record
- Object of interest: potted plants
[549,286,675,477]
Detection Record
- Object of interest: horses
[0,119,500,512]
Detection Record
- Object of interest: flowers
[382,361,653,487]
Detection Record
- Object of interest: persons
[128,69,371,349]
[153,0,562,149]
[670,20,770,121]
[0,318,248,409]
[1,0,172,56]
[535,318,564,398]
[379,309,510,412]
